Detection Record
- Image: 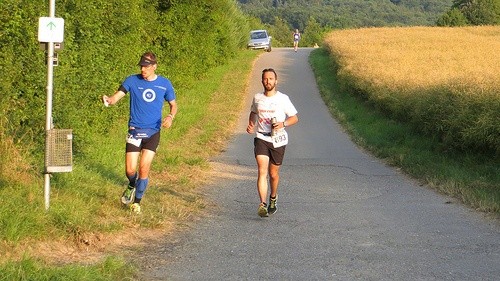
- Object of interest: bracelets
[168,114,174,120]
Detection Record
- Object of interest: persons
[102,52,177,212]
[314,43,319,48]
[246,68,299,217]
[293,29,300,52]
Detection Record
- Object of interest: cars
[247,30,272,52]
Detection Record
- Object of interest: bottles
[271,117,277,136]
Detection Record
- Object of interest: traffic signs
[38,16,65,43]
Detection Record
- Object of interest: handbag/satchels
[128,128,159,139]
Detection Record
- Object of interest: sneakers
[132,201,140,215]
[258,202,268,217]
[268,193,278,214]
[121,185,136,204]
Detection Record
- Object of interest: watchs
[282,122,287,127]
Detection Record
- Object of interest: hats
[137,56,157,66]
